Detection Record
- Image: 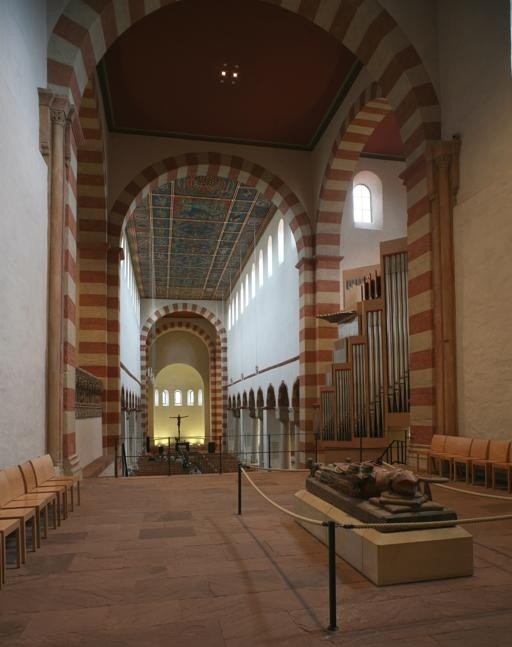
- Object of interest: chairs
[0,451,80,586]
[417,433,512,495]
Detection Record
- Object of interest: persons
[138,444,201,474]
